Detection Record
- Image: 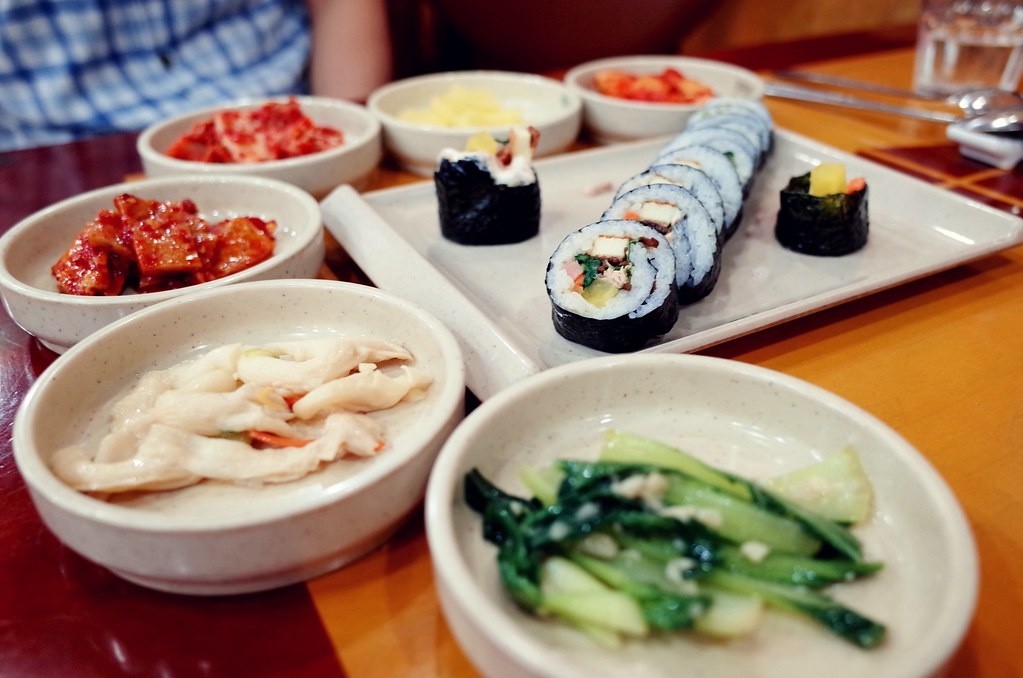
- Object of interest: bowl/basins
[0,173,324,357]
[424,351,979,678]
[492,93,500,98]
[564,55,766,145]
[11,280,465,596]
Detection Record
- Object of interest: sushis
[432,97,869,354]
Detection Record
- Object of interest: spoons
[774,66,1023,115]
[763,81,1023,132]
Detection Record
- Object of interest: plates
[135,95,382,207]
[319,120,1023,403]
[367,70,582,177]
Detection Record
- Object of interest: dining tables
[0,21,1023,678]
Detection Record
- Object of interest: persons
[0,0,392,155]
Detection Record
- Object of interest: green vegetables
[459,436,888,650]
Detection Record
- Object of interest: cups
[909,0,1023,96]
